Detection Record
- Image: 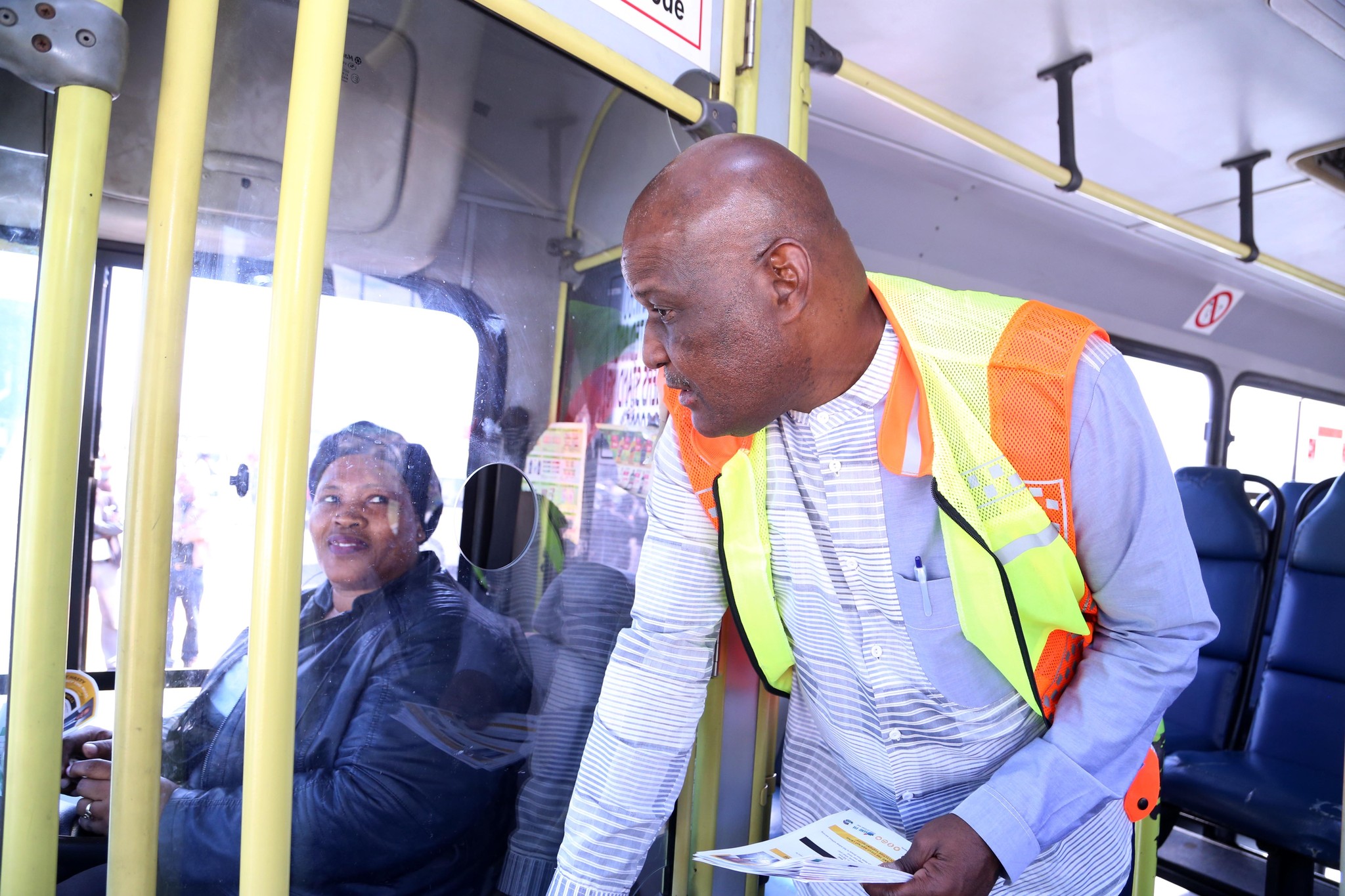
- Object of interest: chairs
[500,566,668,896]
[1162,454,1345,896]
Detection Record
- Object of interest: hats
[307,419,445,546]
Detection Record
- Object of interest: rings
[83,802,96,822]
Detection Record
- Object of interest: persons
[548,132,1223,896]
[57,421,534,896]
